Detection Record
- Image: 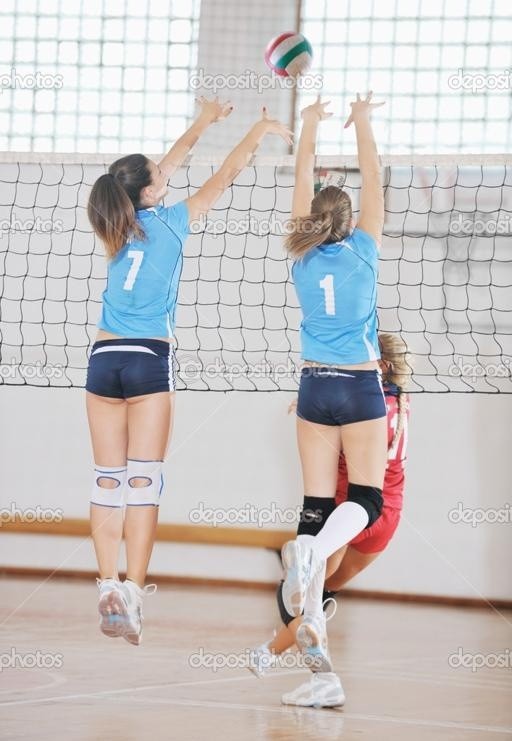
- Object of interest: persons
[80,91,297,647]
[238,330,414,683]
[272,87,391,715]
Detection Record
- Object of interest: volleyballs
[265,32,314,80]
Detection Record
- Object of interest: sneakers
[281,613,344,707]
[96,578,145,646]
[282,534,317,618]
[243,648,276,679]
